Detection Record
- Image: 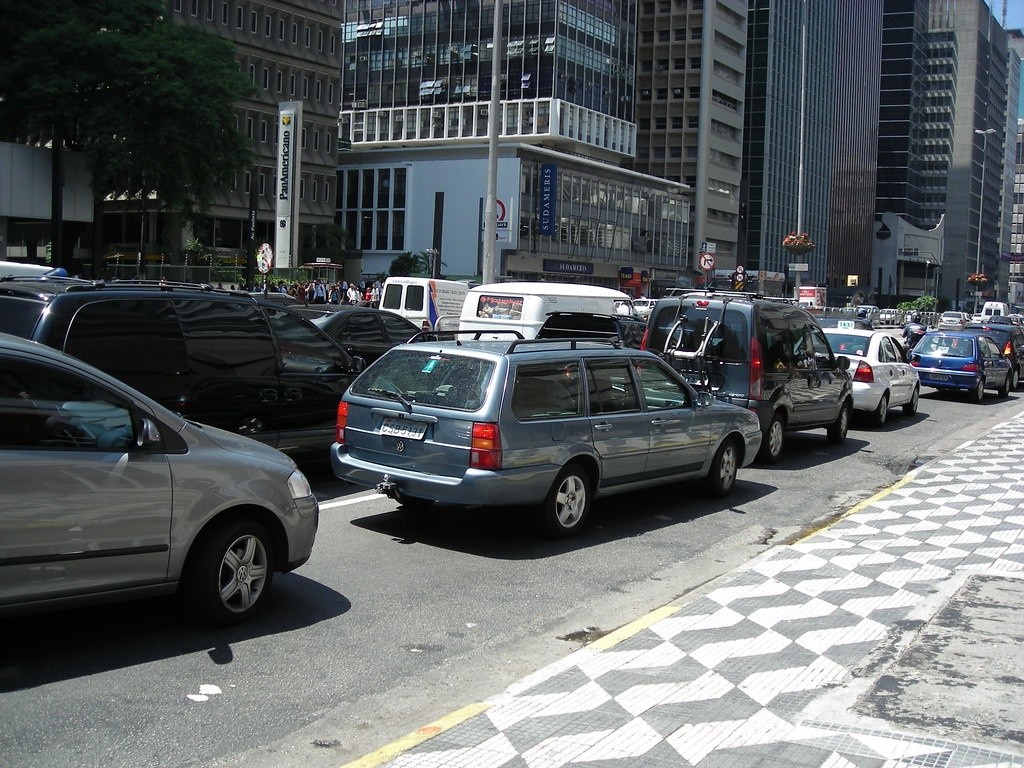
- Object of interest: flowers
[968,274,987,282]
[783,232,816,248]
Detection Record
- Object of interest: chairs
[589,378,621,413]
[513,377,576,417]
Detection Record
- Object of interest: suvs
[0,270,364,453]
[533,310,649,350]
[0,329,322,626]
[330,328,763,540]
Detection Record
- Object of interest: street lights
[426,247,441,277]
[969,128,996,315]
[923,260,932,295]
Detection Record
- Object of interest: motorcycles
[899,324,928,361]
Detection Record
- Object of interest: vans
[640,285,854,463]
[378,275,471,336]
[458,283,640,343]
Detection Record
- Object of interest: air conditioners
[655,65,662,70]
[673,88,681,93]
[481,109,488,115]
[378,112,386,117]
[643,90,648,94]
[432,112,441,118]
[358,102,366,108]
[396,116,403,120]
[338,118,348,124]
[351,103,358,108]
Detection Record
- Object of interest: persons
[269,279,385,309]
[158,276,169,290]
[854,308,874,331]
[214,279,264,294]
[902,311,926,352]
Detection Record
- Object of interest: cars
[794,320,922,428]
[248,289,301,305]
[613,299,661,322]
[852,298,1024,355]
[908,329,1012,403]
[286,301,432,370]
[967,318,1024,393]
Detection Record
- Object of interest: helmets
[911,312,921,324]
[858,308,866,318]
